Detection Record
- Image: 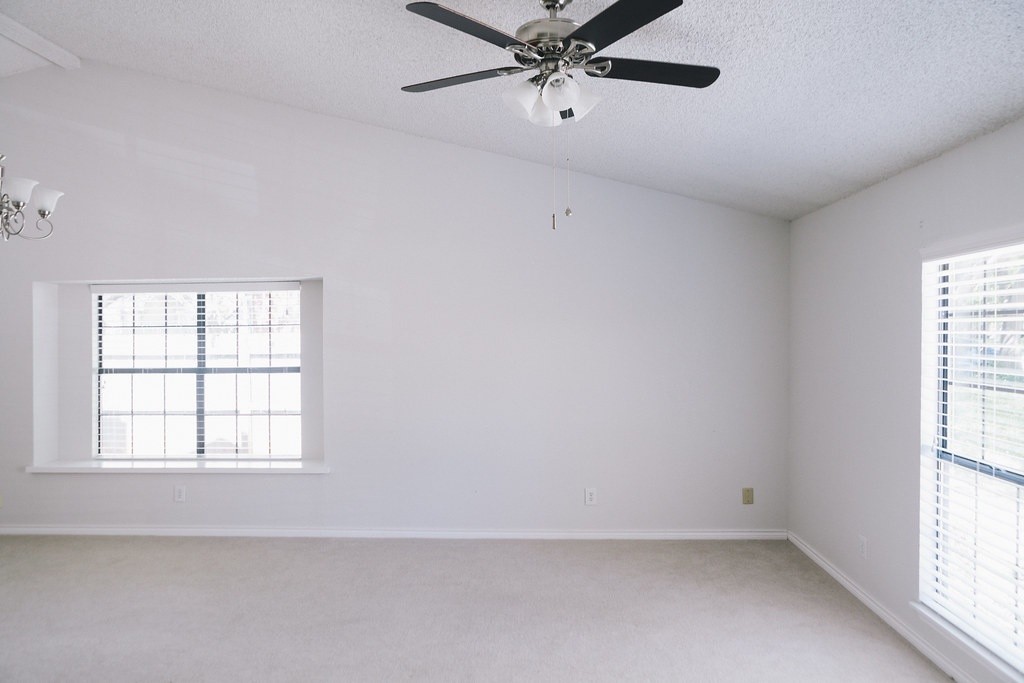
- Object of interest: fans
[402,0,720,95]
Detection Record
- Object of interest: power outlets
[742,487,753,504]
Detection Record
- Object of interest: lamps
[0,154,66,243]
[500,68,602,130]
